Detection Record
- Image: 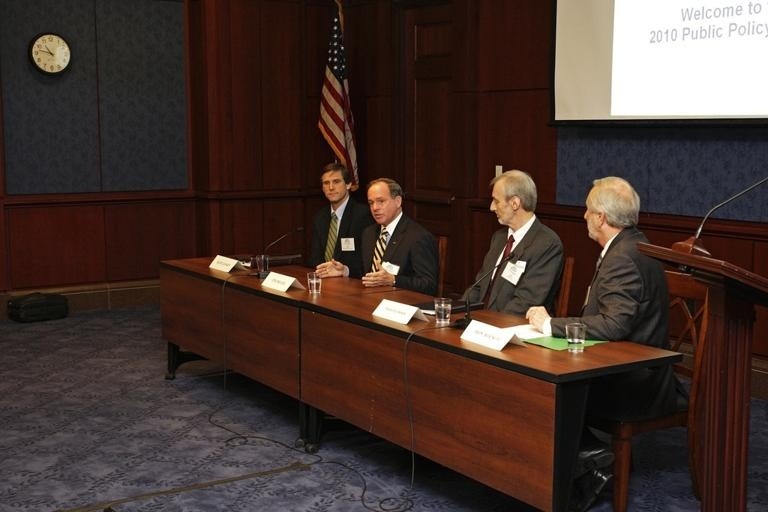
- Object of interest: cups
[256,255,270,273]
[434,298,452,326]
[307,273,322,294]
[566,323,586,352]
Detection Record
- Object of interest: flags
[316,2,361,196]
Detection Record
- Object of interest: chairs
[552,256,574,317]
[608,280,709,512]
[431,236,447,298]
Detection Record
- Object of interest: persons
[526,174,678,510]
[297,161,375,279]
[454,169,563,316]
[313,177,438,295]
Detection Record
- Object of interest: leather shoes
[576,469,616,512]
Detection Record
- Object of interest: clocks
[28,32,73,80]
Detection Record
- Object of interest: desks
[300,289,684,512]
[159,254,402,448]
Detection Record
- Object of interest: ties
[481,234,515,310]
[323,213,338,263]
[369,228,388,273]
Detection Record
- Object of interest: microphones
[257,226,306,279]
[671,177,767,258]
[454,252,515,329]
